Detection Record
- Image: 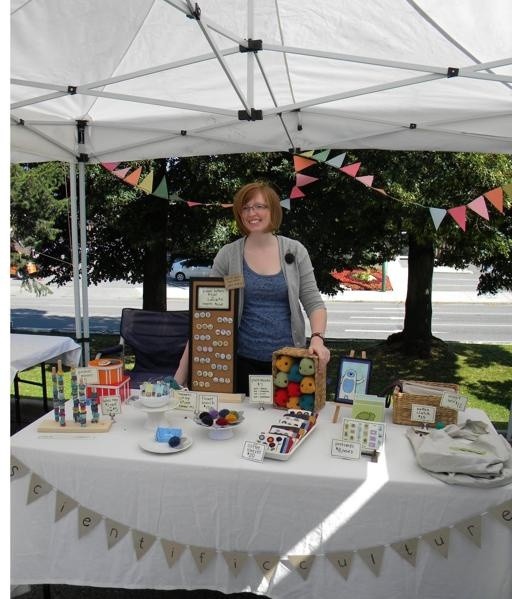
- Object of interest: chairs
[95,308,189,389]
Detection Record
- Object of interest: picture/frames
[341,418,386,456]
[77,368,98,400]
[334,356,373,405]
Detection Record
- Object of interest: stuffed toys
[274,357,315,411]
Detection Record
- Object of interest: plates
[139,434,193,454]
[189,405,246,440]
[124,394,181,412]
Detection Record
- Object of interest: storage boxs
[89,359,123,383]
[392,381,458,428]
[84,376,132,403]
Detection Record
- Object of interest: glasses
[243,203,268,214]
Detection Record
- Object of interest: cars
[170,259,213,280]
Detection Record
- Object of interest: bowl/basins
[139,388,174,408]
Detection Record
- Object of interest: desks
[10,334,81,424]
[10,389,511,599]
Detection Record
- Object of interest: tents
[10,0,512,389]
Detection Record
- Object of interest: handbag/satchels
[406,418,512,486]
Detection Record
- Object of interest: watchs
[309,332,327,341]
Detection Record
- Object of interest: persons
[174,183,330,387]
